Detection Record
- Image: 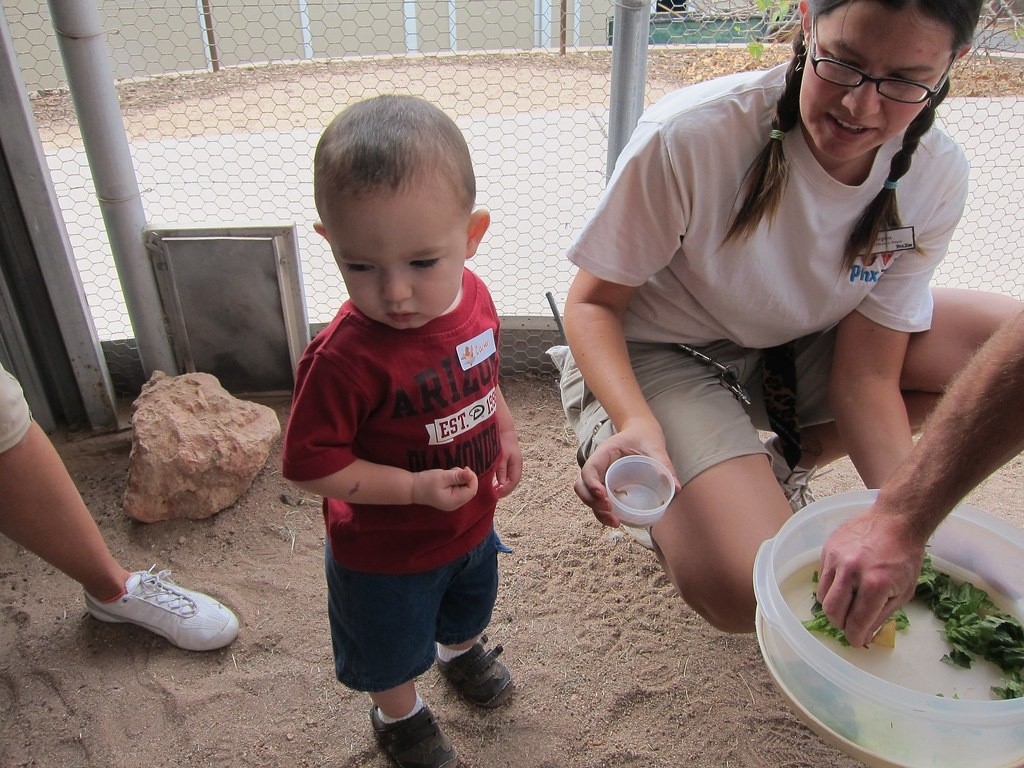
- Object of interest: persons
[279,94,524,768]
[815,310,1024,649]
[549,0,1024,633]
[0,360,240,651]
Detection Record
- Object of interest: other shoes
[434,635,514,709]
[368,702,459,768]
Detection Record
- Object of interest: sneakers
[762,435,833,512]
[83,564,240,650]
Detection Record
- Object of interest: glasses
[809,15,956,104]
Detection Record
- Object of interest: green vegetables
[805,540,1024,701]
